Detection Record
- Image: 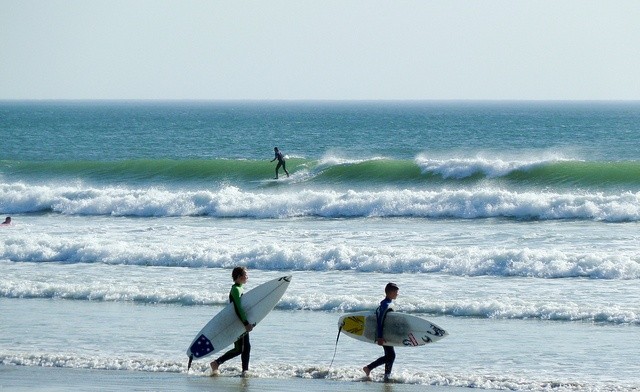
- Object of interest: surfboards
[249,180,280,185]
[338,312,449,347]
[186,274,293,360]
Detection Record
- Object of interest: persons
[210,267,253,372]
[362,282,399,382]
[271,147,290,180]
[2,217,12,225]
